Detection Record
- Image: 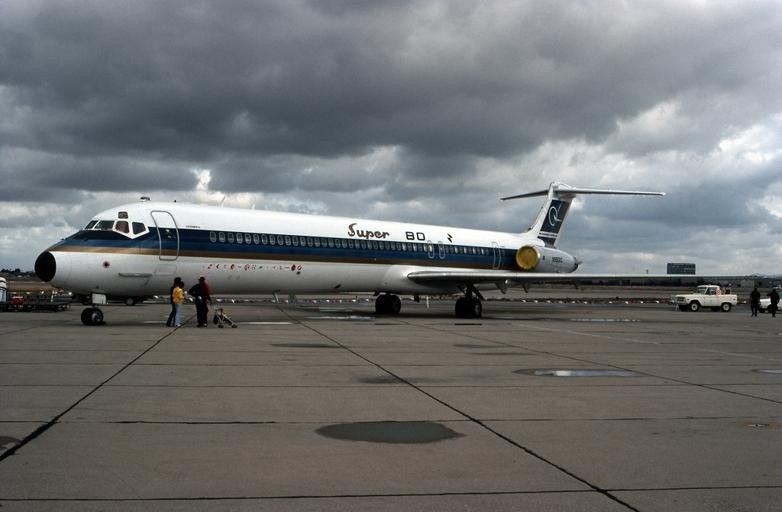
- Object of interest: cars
[757,289,781,314]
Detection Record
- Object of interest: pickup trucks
[673,283,738,313]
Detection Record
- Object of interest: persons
[164,277,182,327]
[766,288,781,318]
[173,282,186,328]
[189,276,214,327]
[748,287,761,318]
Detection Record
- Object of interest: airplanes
[35,179,763,325]
